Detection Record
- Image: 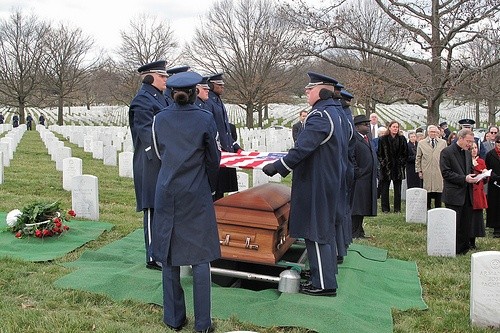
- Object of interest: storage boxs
[212,183,295,266]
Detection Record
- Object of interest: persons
[0,112,4,124]
[129,61,243,271]
[263,71,500,297]
[149,72,221,333]
[39,113,45,126]
[26,113,33,131]
[12,112,19,128]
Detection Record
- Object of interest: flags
[219,148,290,171]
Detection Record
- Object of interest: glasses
[358,123,371,126]
[491,132,497,134]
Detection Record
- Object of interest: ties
[431,139,434,149]
[364,135,368,143]
[372,126,375,140]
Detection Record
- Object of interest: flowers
[0,198,77,239]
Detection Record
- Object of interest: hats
[334,82,345,91]
[166,66,190,75]
[439,122,449,126]
[165,71,203,89]
[137,60,170,77]
[196,76,211,89]
[340,90,354,102]
[304,72,339,88]
[459,119,475,128]
[416,128,424,133]
[210,73,225,84]
[354,115,372,124]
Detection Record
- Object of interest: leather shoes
[301,269,312,281]
[300,284,337,297]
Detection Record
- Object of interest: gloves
[262,163,278,178]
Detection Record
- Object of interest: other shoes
[146,260,162,271]
